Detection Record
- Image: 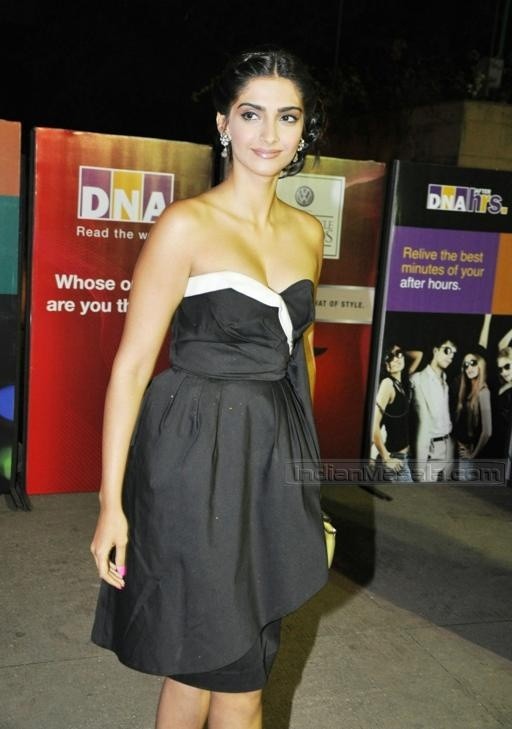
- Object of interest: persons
[365,344,424,486]
[409,332,459,484]
[488,328,511,486]
[452,314,496,483]
[85,50,333,729]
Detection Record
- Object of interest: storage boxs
[401,99,510,171]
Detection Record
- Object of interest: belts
[390,452,410,459]
[433,433,452,442]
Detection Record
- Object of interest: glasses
[497,363,511,373]
[385,350,404,362]
[441,345,454,356]
[464,359,477,368]
